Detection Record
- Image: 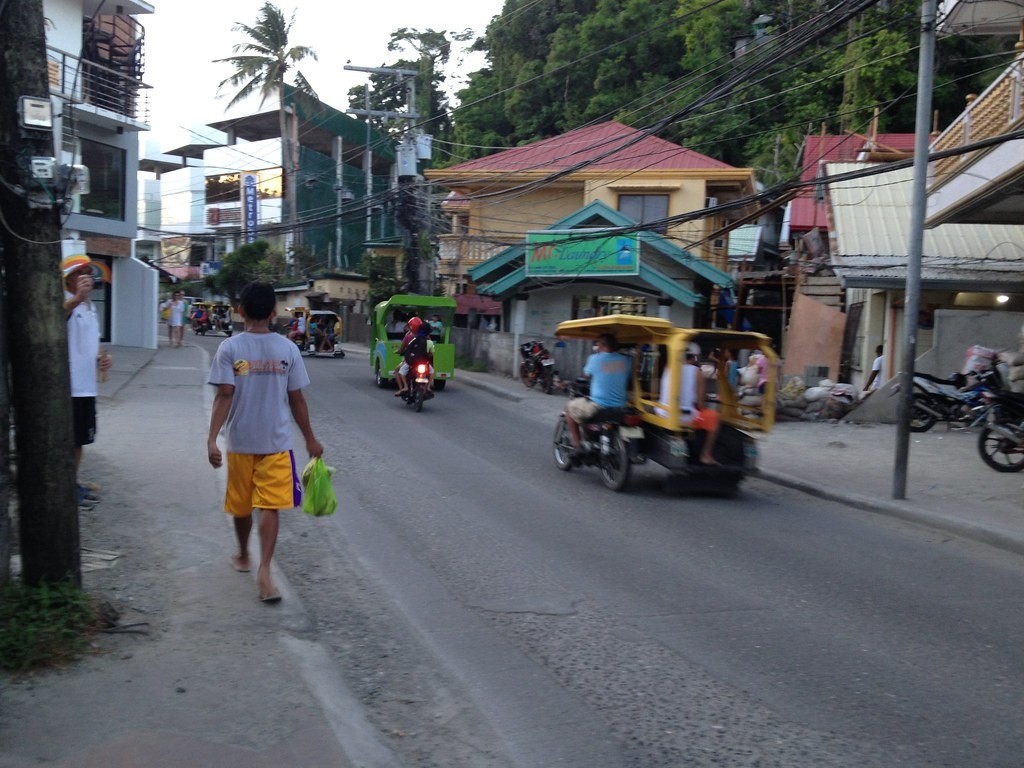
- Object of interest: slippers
[262,588,282,600]
[228,555,251,572]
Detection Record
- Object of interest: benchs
[706,400,763,432]
[627,401,695,433]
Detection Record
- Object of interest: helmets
[408,317,422,334]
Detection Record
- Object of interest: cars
[159,296,203,321]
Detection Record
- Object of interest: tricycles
[366,294,457,390]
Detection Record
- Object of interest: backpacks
[405,338,427,365]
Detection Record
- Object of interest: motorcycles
[282,306,345,359]
[517,340,561,394]
[892,362,1024,433]
[552,314,779,491]
[188,300,233,336]
[392,345,434,412]
[968,391,1024,474]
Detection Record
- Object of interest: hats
[63,255,112,290]
[686,341,701,354]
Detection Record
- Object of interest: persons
[385,310,445,339]
[394,319,437,398]
[161,291,190,347]
[862,344,884,392]
[757,345,781,407]
[283,311,341,354]
[710,346,740,395]
[565,332,633,454]
[63,253,113,513]
[192,305,232,333]
[205,279,324,604]
[657,343,723,466]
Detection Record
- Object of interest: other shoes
[568,447,584,457]
[77,486,101,511]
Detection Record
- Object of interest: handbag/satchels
[301,455,337,516]
